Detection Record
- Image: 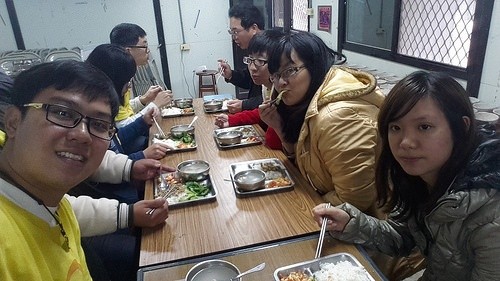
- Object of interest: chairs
[132,51,166,96]
[0,47,84,88]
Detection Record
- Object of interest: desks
[137,231,390,281]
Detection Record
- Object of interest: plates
[153,131,198,151]
[213,125,263,149]
[203,99,229,113]
[161,105,195,118]
[274,252,375,281]
[229,157,295,195]
[154,170,218,207]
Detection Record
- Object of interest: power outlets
[180,44,190,51]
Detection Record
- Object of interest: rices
[155,193,189,204]
[153,137,177,149]
[161,106,182,116]
[312,260,371,281]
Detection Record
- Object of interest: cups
[185,259,242,281]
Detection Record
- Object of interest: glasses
[128,45,148,52]
[243,57,269,67]
[270,65,307,83]
[18,103,119,141]
[228,26,249,36]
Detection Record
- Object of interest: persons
[84,22,173,152]
[312,68,500,281]
[214,27,289,152]
[0,69,174,266]
[258,31,432,281]
[216,2,268,113]
[0,57,131,281]
[82,43,173,204]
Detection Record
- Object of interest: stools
[196,70,219,98]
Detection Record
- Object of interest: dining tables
[139,94,329,267]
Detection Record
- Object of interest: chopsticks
[314,202,331,258]
[216,59,228,83]
[270,97,279,106]
[145,184,177,214]
[152,116,165,136]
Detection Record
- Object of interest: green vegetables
[177,131,192,143]
[181,180,210,201]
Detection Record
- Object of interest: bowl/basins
[170,124,195,140]
[234,168,266,191]
[174,98,193,108]
[216,131,243,146]
[177,159,210,182]
[203,100,223,111]
